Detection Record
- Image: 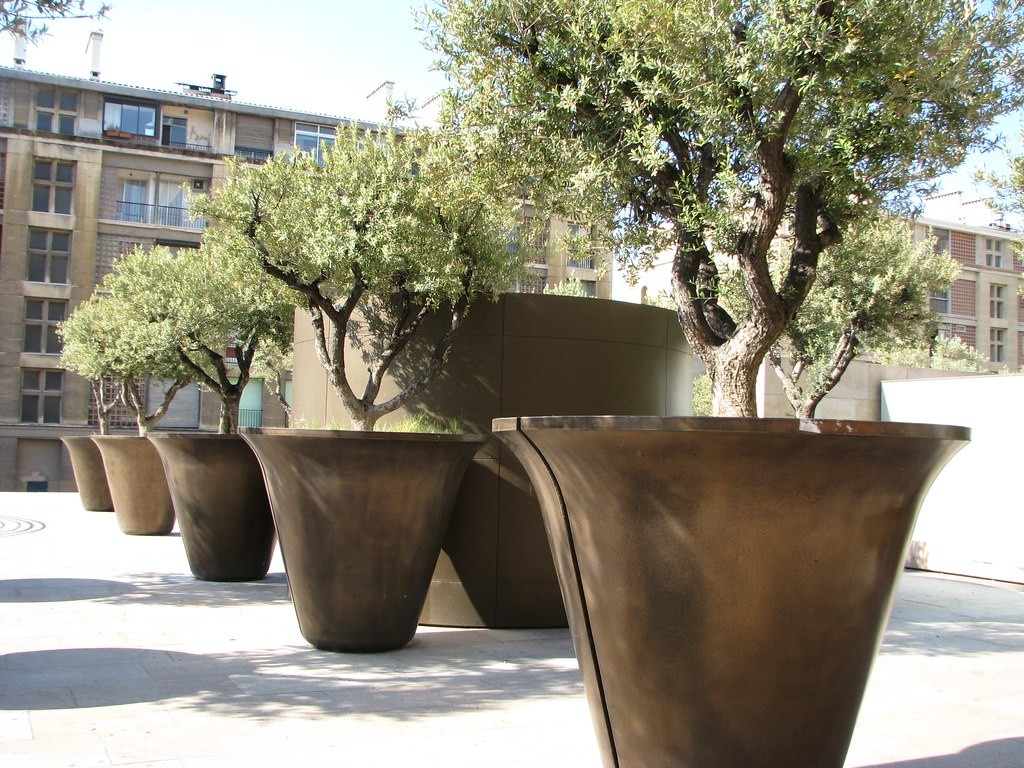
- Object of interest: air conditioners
[191,178,208,193]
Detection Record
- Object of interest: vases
[119,132,131,139]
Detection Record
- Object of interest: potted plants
[107,123,119,136]
[425,0,1024,768]
[177,94,490,651]
[55,300,112,511]
[89,243,176,534]
[144,227,279,582]
